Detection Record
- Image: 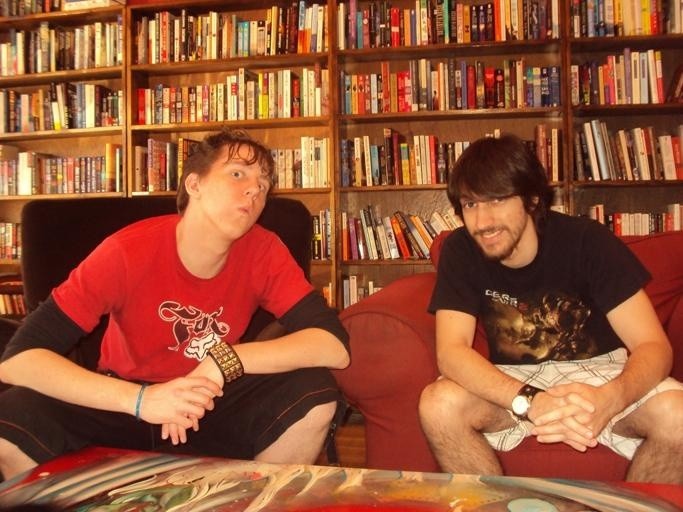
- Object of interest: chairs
[328,229,682,482]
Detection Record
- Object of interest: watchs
[511,382,546,420]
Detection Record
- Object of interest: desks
[1,445,683,512]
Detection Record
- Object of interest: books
[312,209,332,308]
[1,2,125,316]
[132,0,330,193]
[569,2,683,237]
[337,1,560,311]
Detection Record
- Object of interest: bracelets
[132,381,150,422]
[206,340,245,384]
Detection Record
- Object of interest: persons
[418,133,683,486]
[0,127,353,483]
